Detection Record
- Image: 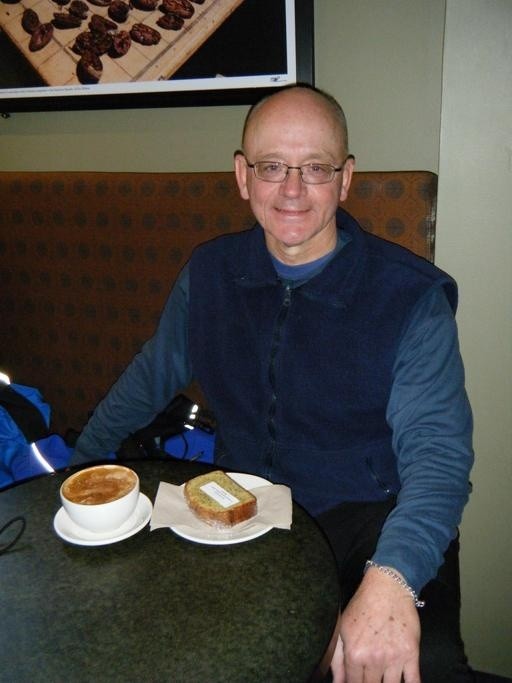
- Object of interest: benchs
[1,171,437,509]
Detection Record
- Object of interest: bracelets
[362,560,425,608]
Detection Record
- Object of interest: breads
[182,467,259,529]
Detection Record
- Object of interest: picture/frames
[0,0,316,113]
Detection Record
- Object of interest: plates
[170,472,279,546]
[53,492,153,547]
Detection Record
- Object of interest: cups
[59,464,140,532]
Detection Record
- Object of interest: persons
[73,84,472,683]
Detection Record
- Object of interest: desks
[0,454,342,683]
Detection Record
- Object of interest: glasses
[244,158,349,185]
